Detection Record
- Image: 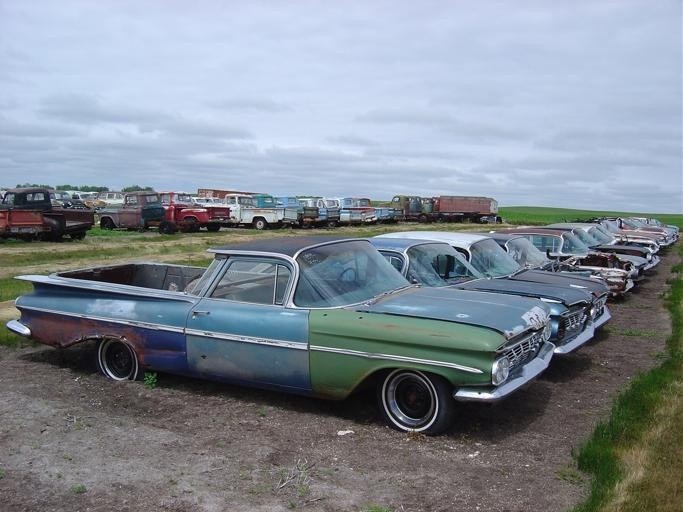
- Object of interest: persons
[60,198,74,209]
